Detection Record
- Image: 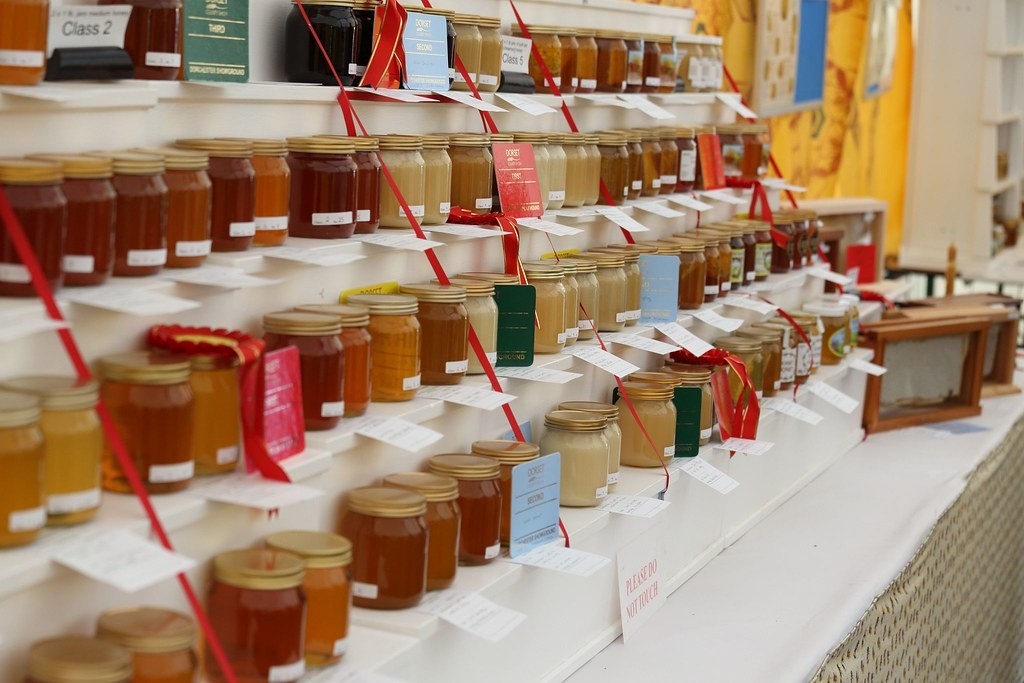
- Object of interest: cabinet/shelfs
[0,0,874,683]
[897,0,1024,277]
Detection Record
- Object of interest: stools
[818,226,849,281]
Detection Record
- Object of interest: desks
[781,199,888,282]
